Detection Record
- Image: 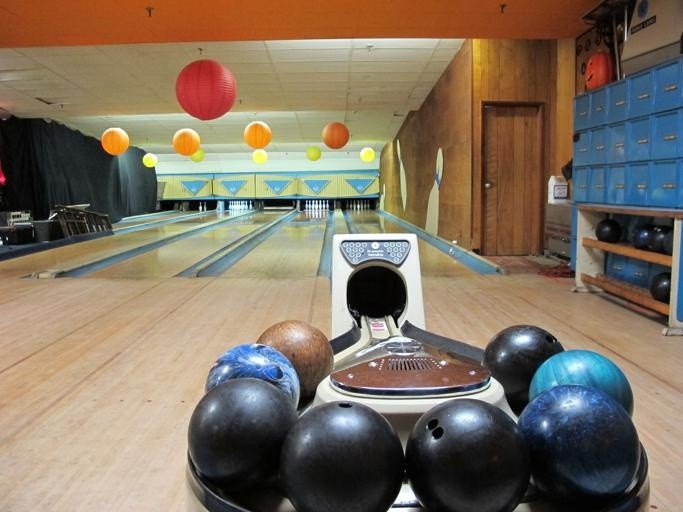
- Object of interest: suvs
[547,176,568,204]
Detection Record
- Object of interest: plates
[572,57,682,334]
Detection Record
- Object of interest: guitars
[305,145,321,161]
[172,128,200,156]
[142,153,158,168]
[175,60,238,121]
[101,127,128,155]
[190,149,204,162]
[252,150,267,163]
[360,147,376,163]
[322,123,350,149]
[244,120,271,149]
